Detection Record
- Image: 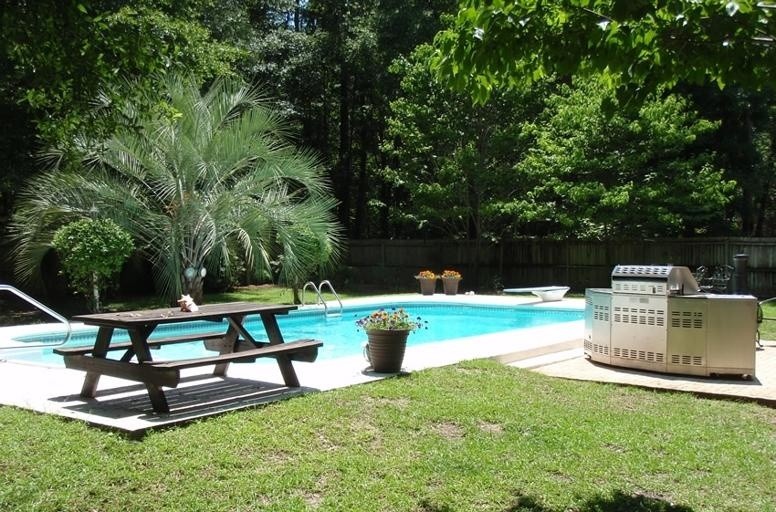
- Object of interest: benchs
[141,338,324,388]
[54,332,225,368]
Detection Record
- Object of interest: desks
[71,303,300,416]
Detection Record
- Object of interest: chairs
[692,265,735,293]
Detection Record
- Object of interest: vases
[441,276,463,295]
[366,328,410,374]
[419,278,436,295]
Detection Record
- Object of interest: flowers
[414,270,440,280]
[353,307,428,333]
[443,270,461,278]
